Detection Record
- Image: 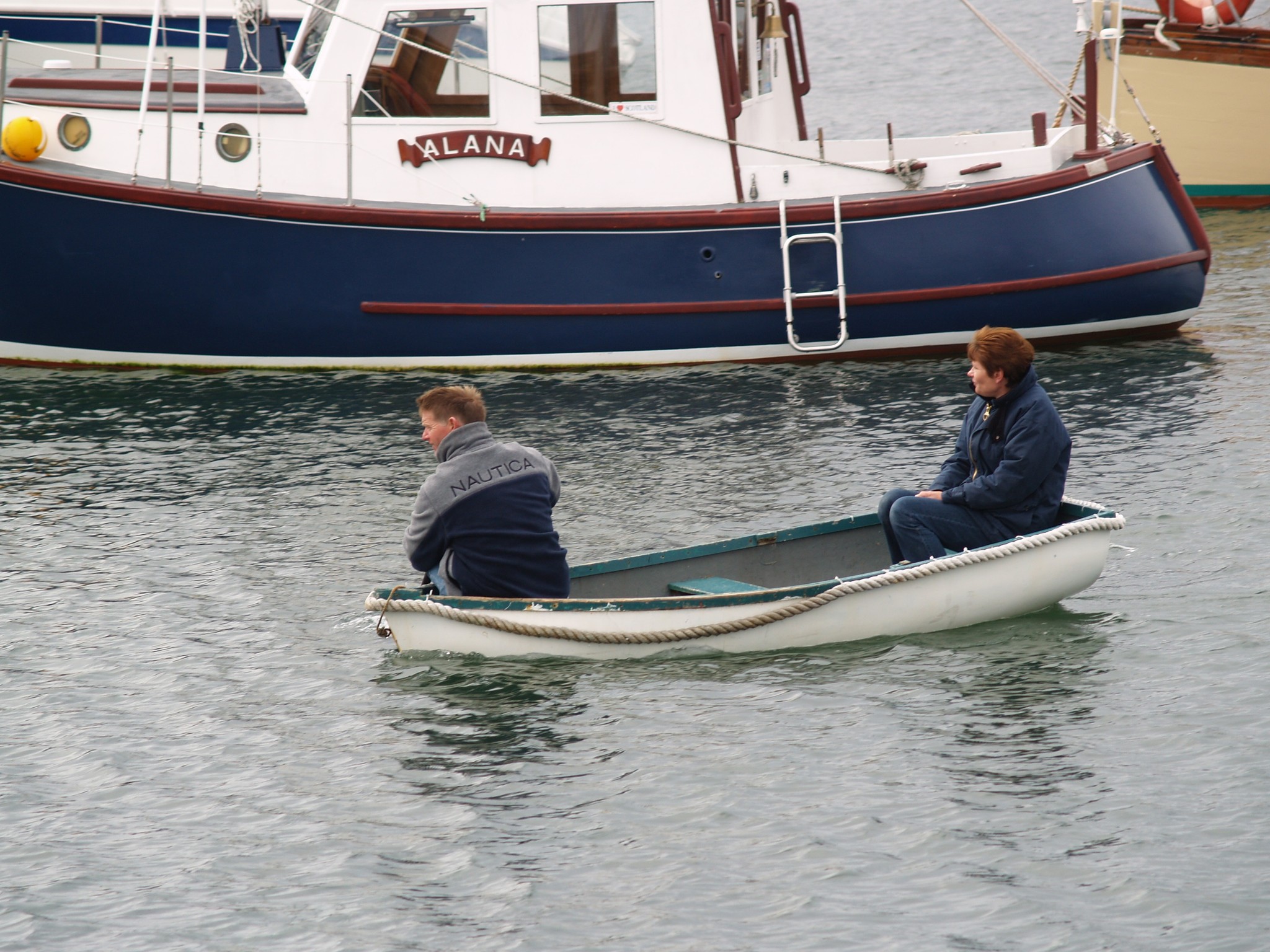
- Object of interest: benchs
[668,576,768,594]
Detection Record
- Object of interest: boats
[0,0,1209,383]
[1070,0,1269,209]
[365,490,1128,661]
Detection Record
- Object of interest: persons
[880,326,1071,565]
[403,384,570,600]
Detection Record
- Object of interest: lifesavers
[1155,0,1255,27]
